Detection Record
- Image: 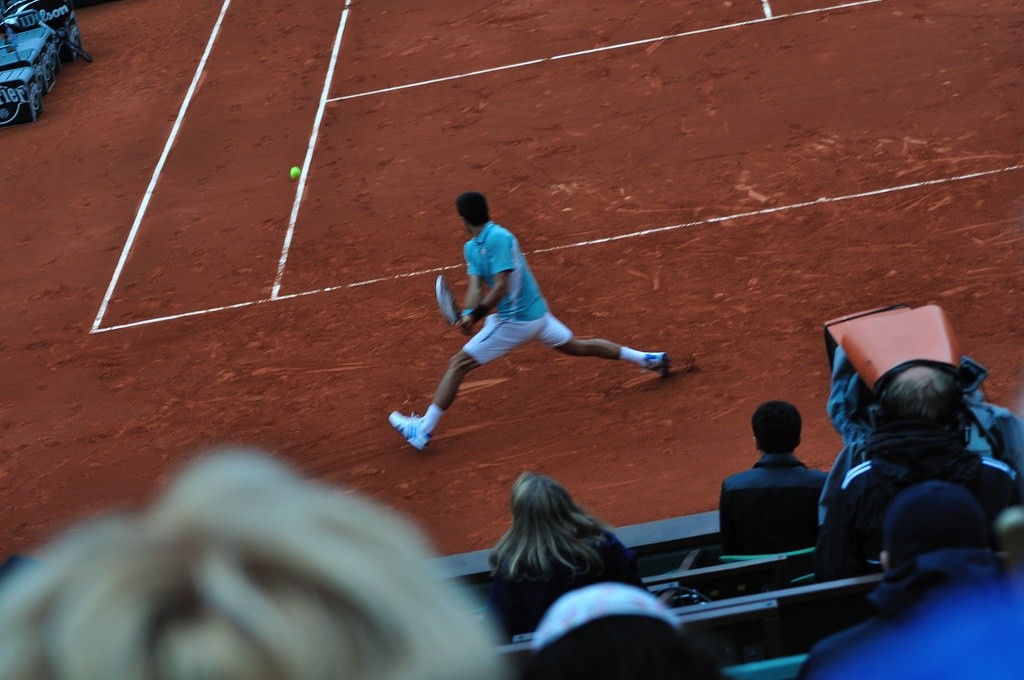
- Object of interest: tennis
[290,166,301,180]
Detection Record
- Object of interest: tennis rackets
[435,275,461,326]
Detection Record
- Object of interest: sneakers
[644,351,669,376]
[387,411,430,449]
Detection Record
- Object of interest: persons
[388,191,671,453]
[489,344,1024,680]
[0,444,526,680]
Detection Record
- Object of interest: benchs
[421,508,893,680]
